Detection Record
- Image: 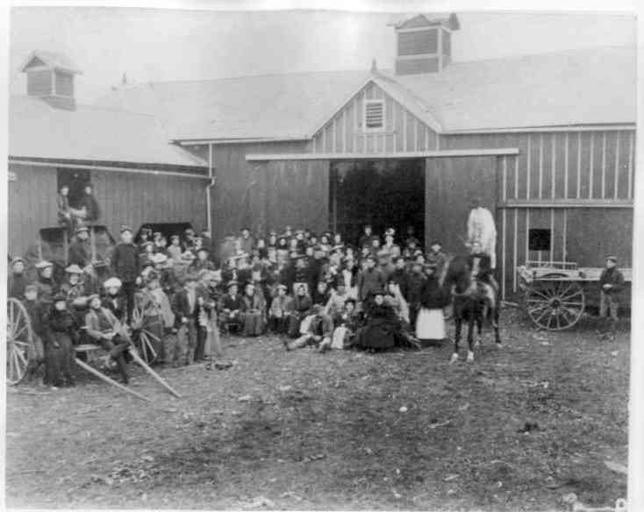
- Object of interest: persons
[7,225,452,383]
[57,183,100,234]
[464,196,497,277]
[598,255,625,321]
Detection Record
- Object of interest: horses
[436,254,502,365]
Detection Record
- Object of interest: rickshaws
[518,261,632,332]
[7,286,181,402]
